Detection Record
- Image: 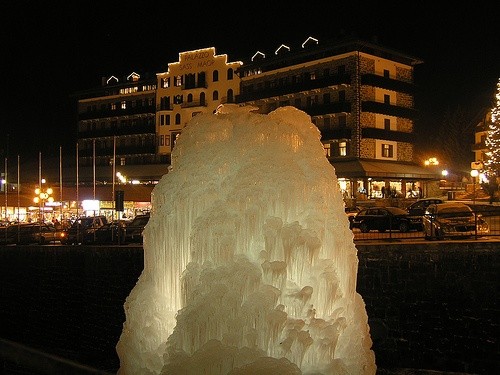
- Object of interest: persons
[52,217,55,223]
[408,188,413,198]
[54,219,60,226]
[418,187,422,199]
[381,186,396,198]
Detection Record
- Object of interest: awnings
[40,207,53,213]
[330,160,439,177]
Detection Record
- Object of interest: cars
[0,213,150,245]
[345,207,361,230]
[422,202,490,240]
[353,206,407,233]
[396,199,444,233]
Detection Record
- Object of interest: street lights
[441,169,449,196]
[470,170,478,240]
[368,177,374,200]
